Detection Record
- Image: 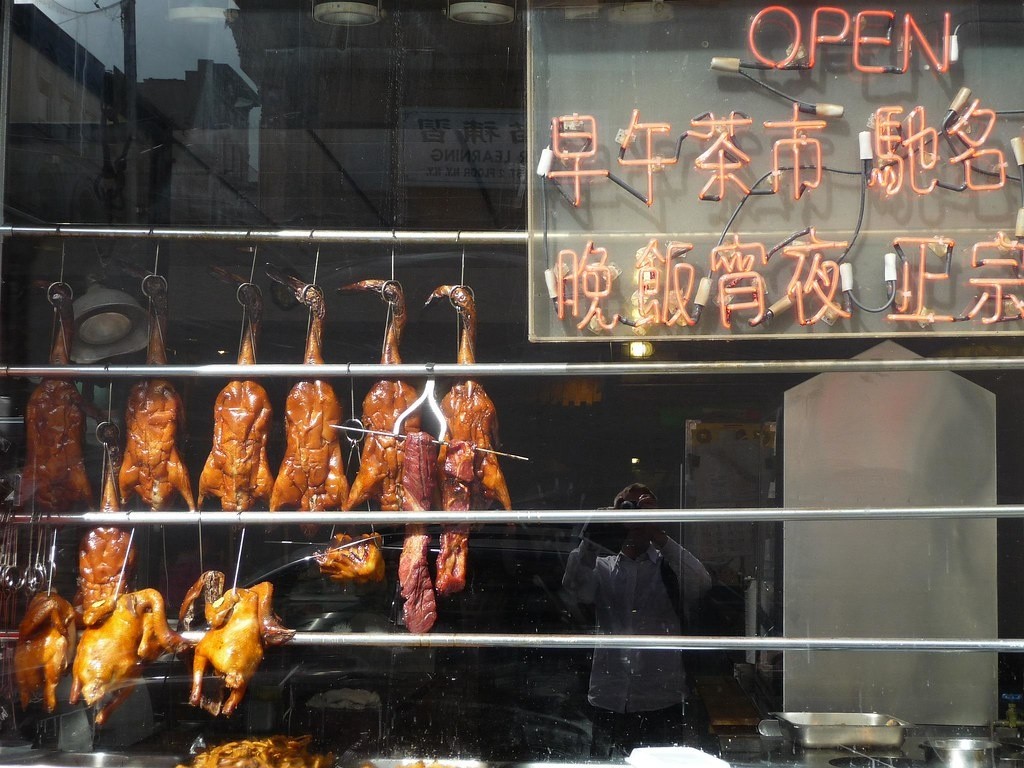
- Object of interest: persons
[562,483,712,760]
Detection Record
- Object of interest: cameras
[620,501,639,510]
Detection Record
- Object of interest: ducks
[12,267,515,718]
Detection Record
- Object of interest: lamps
[607,0,675,24]
[166,0,233,23]
[312,0,387,27]
[442,0,517,26]
[72,272,152,365]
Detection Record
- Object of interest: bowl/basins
[919,737,1003,768]
